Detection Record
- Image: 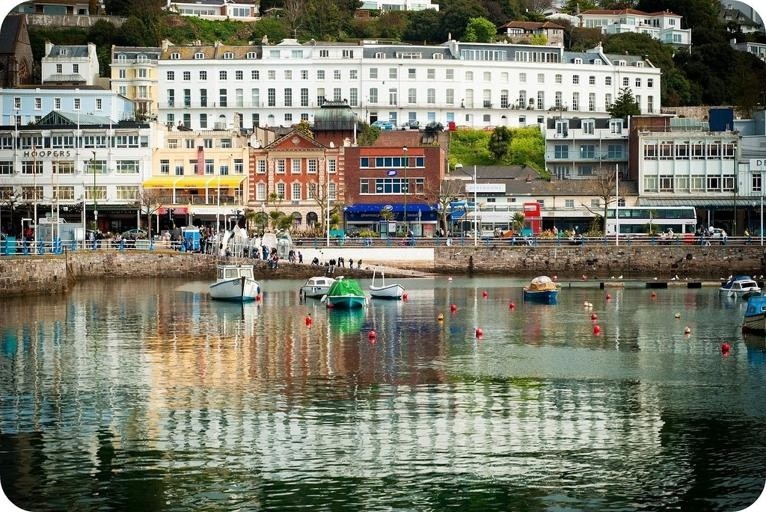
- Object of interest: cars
[123,228,147,239]
[709,228,727,245]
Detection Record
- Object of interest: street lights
[459,167,477,246]
[92,150,98,231]
[403,145,407,235]
[733,147,737,236]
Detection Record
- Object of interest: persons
[243,246,362,268]
[172,225,232,255]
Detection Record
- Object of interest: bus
[606,207,699,240]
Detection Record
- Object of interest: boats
[326,277,365,310]
[210,265,261,303]
[369,268,405,300]
[524,287,558,304]
[719,275,761,293]
[743,296,766,337]
[300,276,335,295]
